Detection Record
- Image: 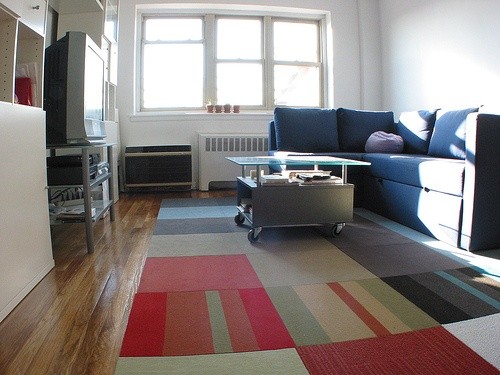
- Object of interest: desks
[226,156,371,243]
[46,143,115,255]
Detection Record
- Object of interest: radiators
[123,144,192,192]
[199,133,269,192]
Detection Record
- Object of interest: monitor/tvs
[42,30,107,146]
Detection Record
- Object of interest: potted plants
[206,98,240,113]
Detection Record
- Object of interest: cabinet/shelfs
[0,0,120,324]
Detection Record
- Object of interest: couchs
[269,107,500,253]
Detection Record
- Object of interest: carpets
[115,198,500,375]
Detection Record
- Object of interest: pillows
[336,108,394,151]
[396,109,440,155]
[365,130,404,153]
[428,108,478,159]
[273,107,338,153]
